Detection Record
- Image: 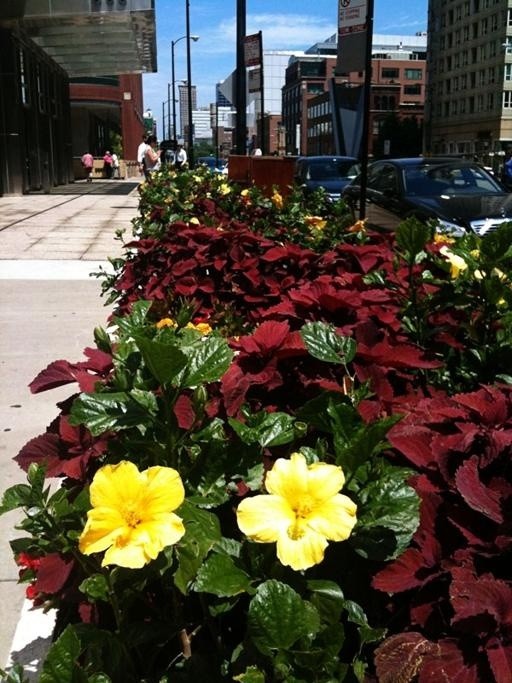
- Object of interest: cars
[342,156,511,242]
[292,155,361,216]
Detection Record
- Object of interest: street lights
[162,79,188,146]
[172,34,199,148]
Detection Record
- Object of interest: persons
[137,131,151,166]
[156,138,187,168]
[81,150,94,182]
[102,150,113,178]
[111,150,121,179]
[144,134,161,170]
